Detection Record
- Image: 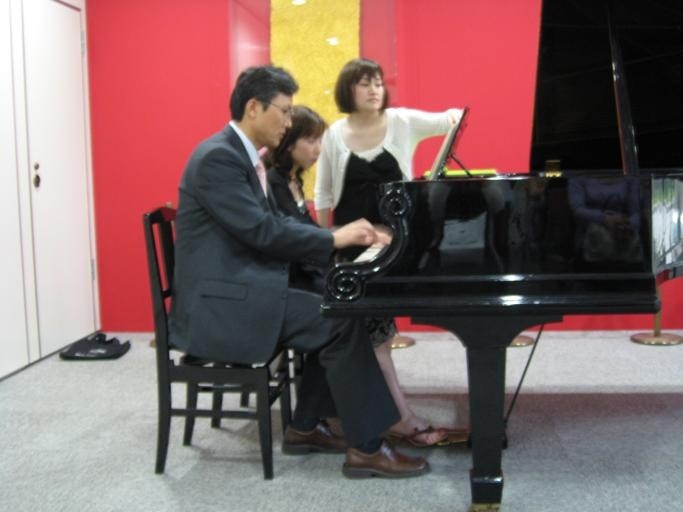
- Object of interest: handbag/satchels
[60,333,132,360]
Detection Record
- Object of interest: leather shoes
[343,442,431,478]
[281,423,351,454]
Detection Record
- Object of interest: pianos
[320,18,661,511]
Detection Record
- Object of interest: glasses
[261,99,295,120]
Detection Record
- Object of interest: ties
[255,161,268,198]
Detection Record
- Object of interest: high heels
[384,418,452,449]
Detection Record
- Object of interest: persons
[260,105,448,450]
[166,62,434,480]
[313,58,469,264]
[412,175,647,273]
